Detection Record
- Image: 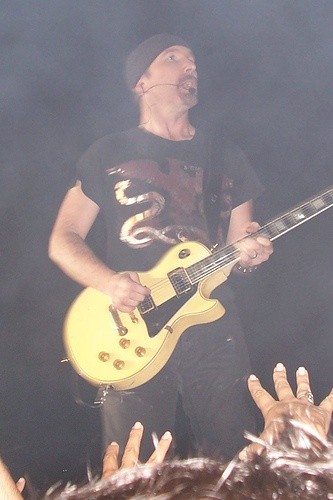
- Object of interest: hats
[124,34,194,91]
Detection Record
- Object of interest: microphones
[137,83,196,94]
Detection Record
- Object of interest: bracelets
[233,262,260,274]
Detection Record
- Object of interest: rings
[297,392,314,404]
[251,253,257,259]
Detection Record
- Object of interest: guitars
[62,183,333,394]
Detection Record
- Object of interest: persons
[46,33,274,465]
[1,363,332,500]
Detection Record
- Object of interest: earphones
[140,82,145,90]
